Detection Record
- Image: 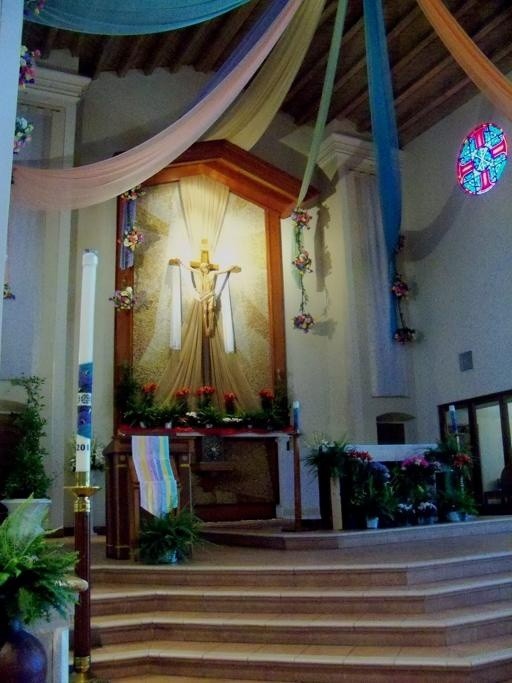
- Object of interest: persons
[175,257,241,337]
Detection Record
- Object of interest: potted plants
[0,374,84,683]
[133,500,224,565]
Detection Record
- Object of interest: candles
[293,400,299,429]
[449,405,458,433]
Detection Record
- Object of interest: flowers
[300,429,480,520]
[109,184,150,311]
[119,382,293,428]
[0,0,46,301]
[292,207,315,333]
[390,232,416,344]
